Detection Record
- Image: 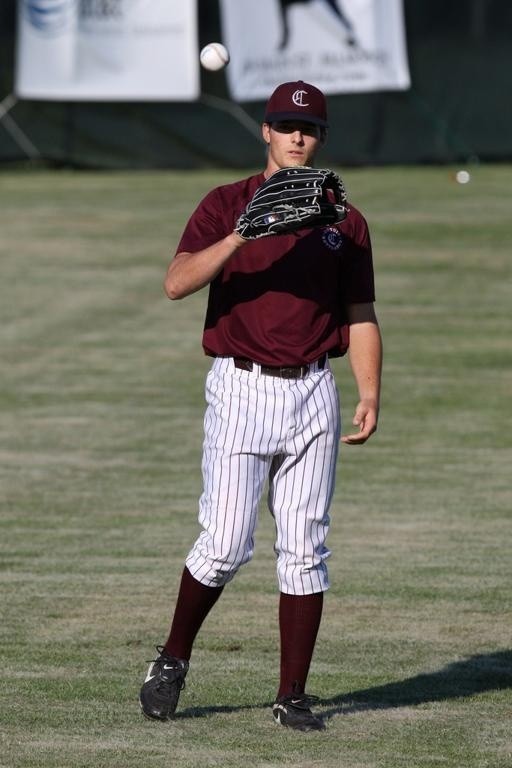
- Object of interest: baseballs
[456,171,470,184]
[199,43,230,73]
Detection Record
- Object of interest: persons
[139,81,383,734]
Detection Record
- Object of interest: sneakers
[272,694,326,733]
[137,647,189,721]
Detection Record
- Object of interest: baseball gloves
[233,165,349,241]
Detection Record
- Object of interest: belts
[234,356,326,379]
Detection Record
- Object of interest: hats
[262,80,327,129]
